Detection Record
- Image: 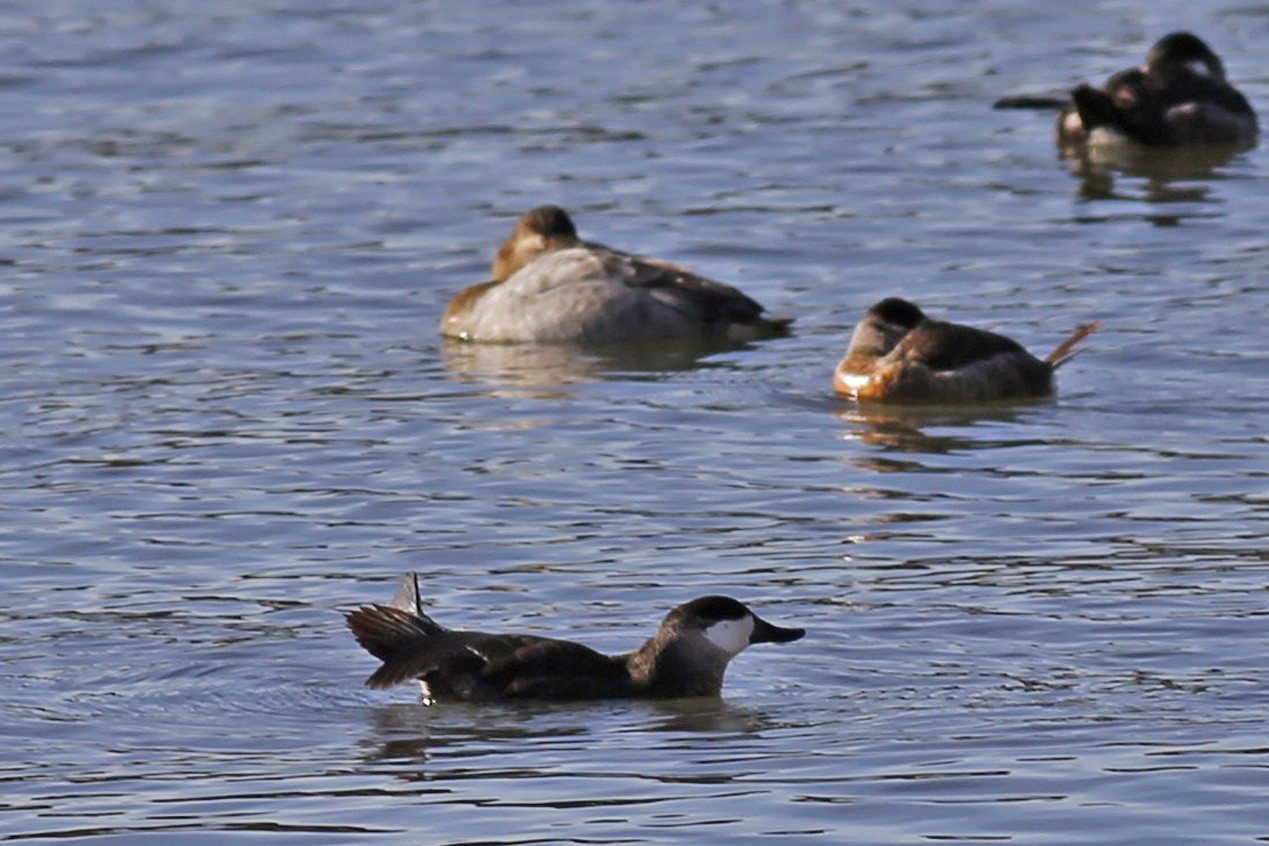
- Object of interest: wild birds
[1055,31,1256,146]
[835,295,1102,399]
[438,204,797,337]
[344,573,805,700]
